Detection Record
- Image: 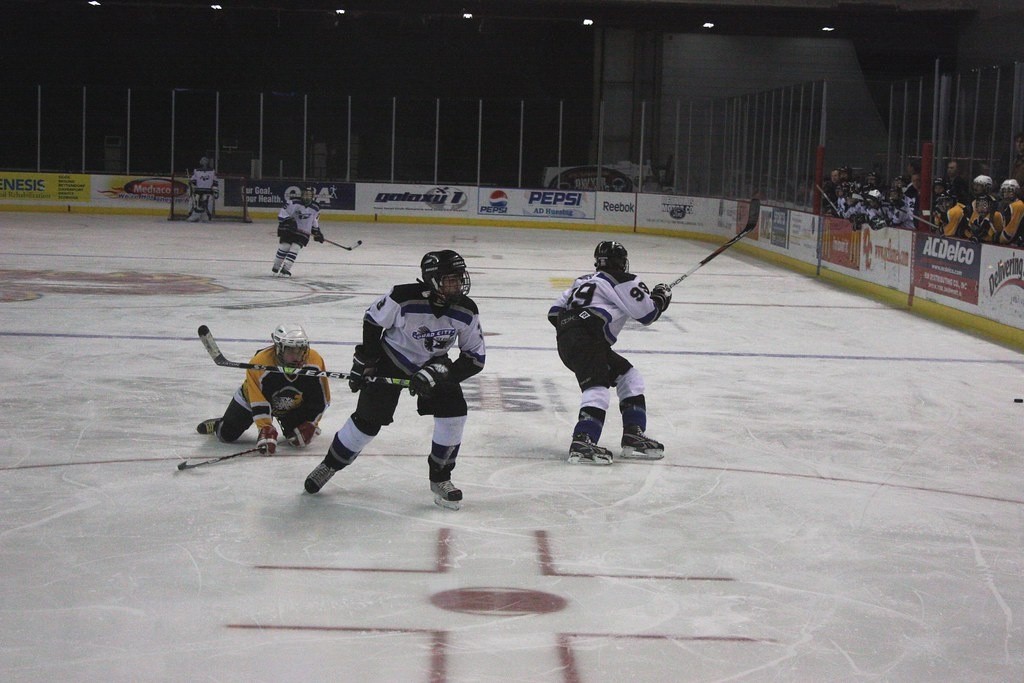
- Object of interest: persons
[547,240,672,465]
[302,249,485,511]
[822,135,1024,248]
[195,322,331,454]
[186,156,220,222]
[272,187,324,278]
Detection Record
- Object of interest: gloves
[288,219,296,232]
[286,421,316,447]
[348,344,381,391]
[257,426,278,454]
[409,361,448,395]
[314,231,323,243]
[650,283,672,311]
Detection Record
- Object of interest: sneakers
[430,479,463,510]
[567,433,613,465]
[272,263,281,276]
[197,418,222,434]
[619,424,664,459]
[278,267,291,278]
[302,460,336,495]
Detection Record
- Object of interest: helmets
[840,166,1019,213]
[199,157,209,165]
[594,240,629,273]
[273,322,310,368]
[420,250,470,303]
[301,185,314,203]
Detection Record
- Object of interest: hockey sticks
[895,206,940,228]
[177,436,295,470]
[1014,399,1023,404]
[816,184,839,211]
[297,228,362,251]
[851,193,887,218]
[197,325,411,388]
[666,198,760,291]
[186,168,194,209]
